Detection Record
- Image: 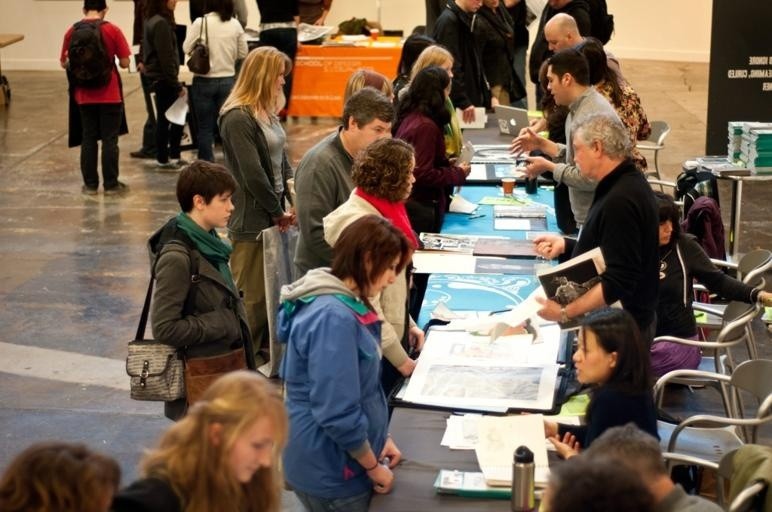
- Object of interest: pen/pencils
[469,215,486,219]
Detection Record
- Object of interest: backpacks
[66,18,116,89]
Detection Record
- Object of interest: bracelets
[758,291,765,307]
[364,459,379,470]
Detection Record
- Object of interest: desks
[363,108,582,512]
[0,31,24,105]
[683,153,771,260]
[238,28,420,124]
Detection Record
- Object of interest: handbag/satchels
[126,341,250,410]
[188,42,210,72]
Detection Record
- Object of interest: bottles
[511,445,535,512]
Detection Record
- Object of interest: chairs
[692,244,772,369]
[647,354,772,471]
[648,276,772,442]
[657,443,772,512]
[643,170,724,262]
[632,116,674,197]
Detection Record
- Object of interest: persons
[217,45,298,370]
[510,13,661,389]
[110,370,289,511]
[581,422,727,511]
[273,214,413,511]
[425,1,615,133]
[294,35,473,398]
[521,306,661,459]
[148,160,258,421]
[650,191,772,383]
[60,0,332,194]
[0,438,122,511]
[539,454,661,511]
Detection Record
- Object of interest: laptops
[495,105,544,136]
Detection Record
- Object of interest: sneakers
[82,184,97,195]
[104,182,126,194]
[123,144,197,172]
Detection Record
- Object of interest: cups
[501,177,517,196]
[369,28,379,40]
[525,177,538,194]
